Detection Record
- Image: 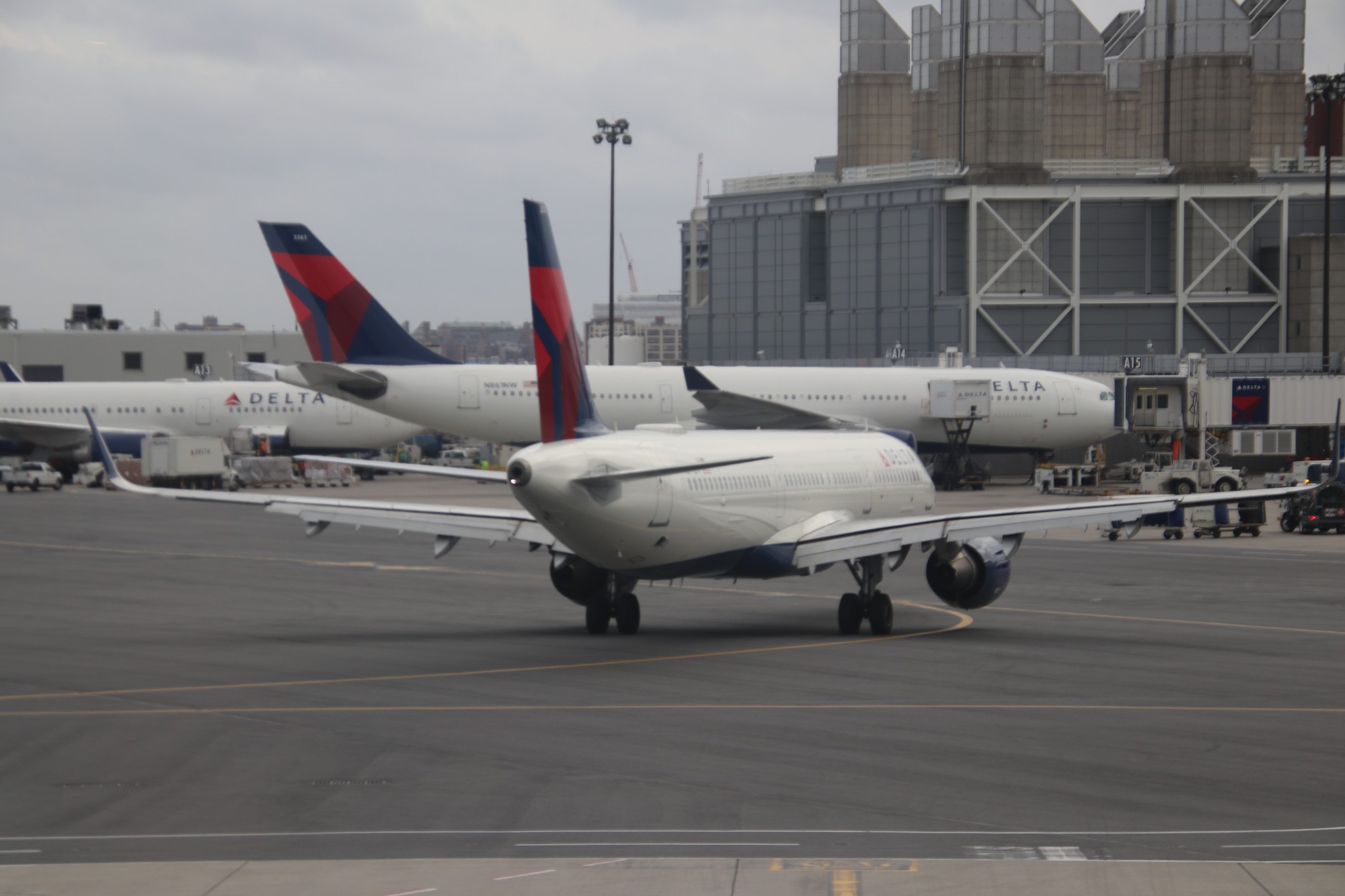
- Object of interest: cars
[1280,479,1345,536]
[433,448,475,469]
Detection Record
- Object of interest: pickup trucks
[0,460,64,491]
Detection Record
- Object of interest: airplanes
[0,197,1345,637]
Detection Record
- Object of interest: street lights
[591,117,632,365]
